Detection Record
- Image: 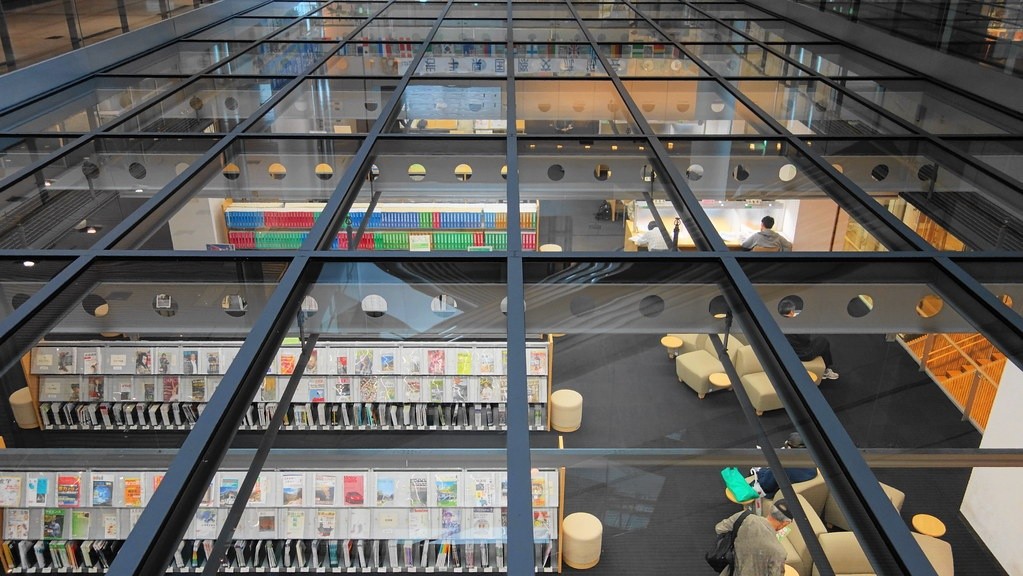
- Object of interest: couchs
[667,333,827,416]
[746,467,955,576]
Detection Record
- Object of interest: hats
[787,432,807,449]
[774,498,794,520]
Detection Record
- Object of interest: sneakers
[822,368,839,380]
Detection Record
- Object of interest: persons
[481,379,492,400]
[70,384,79,400]
[636,221,681,253]
[93,378,103,401]
[307,351,317,370]
[209,355,216,369]
[45,520,62,538]
[161,353,169,372]
[741,215,793,253]
[759,429,819,501]
[186,354,198,374]
[716,499,795,576]
[443,513,460,536]
[137,353,149,373]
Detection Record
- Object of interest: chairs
[751,246,779,251]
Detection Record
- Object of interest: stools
[562,512,603,570]
[551,389,584,432]
[8,387,40,429]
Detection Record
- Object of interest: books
[3,473,559,568]
[30,349,547,425]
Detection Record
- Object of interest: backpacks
[705,509,752,576]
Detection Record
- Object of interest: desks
[806,371,817,383]
[726,486,756,511]
[539,243,562,253]
[708,372,733,392]
[782,563,800,575]
[661,336,683,358]
[912,514,947,537]
[625,219,791,252]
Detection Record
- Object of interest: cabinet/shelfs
[221,197,539,253]
